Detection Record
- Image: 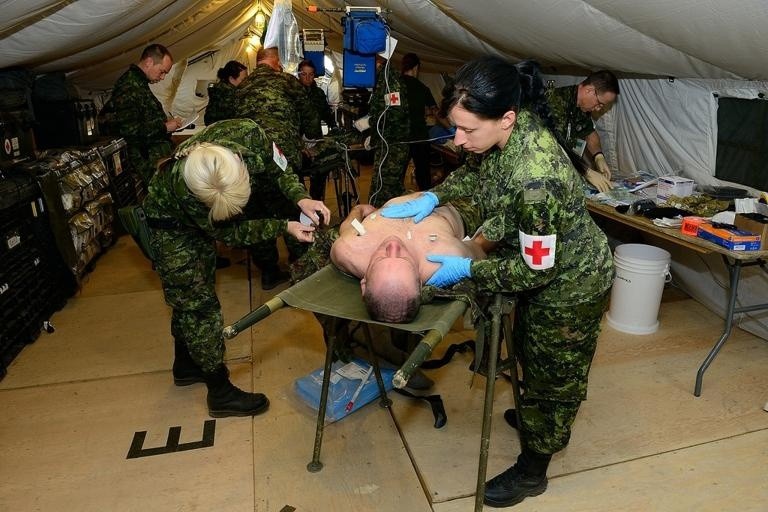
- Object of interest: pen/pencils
[168,111,174,119]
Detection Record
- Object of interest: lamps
[254,1,265,29]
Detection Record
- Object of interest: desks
[580,165,768,397]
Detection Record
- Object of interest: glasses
[594,89,607,113]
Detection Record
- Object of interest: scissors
[702,219,737,230]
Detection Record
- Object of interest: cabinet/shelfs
[1,178,72,382]
[88,137,140,238]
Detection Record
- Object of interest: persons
[142,118,330,418]
[379,55,615,508]
[112,44,232,270]
[544,70,621,193]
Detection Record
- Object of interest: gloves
[381,189,441,224]
[425,256,473,288]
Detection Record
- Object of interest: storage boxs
[733,210,767,253]
[655,173,695,209]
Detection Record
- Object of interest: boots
[258,263,291,290]
[171,339,230,386]
[503,406,525,432]
[216,255,232,268]
[483,448,553,508]
[205,366,270,418]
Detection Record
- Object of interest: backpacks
[339,319,436,392]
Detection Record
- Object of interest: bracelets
[592,152,604,161]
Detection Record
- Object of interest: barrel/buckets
[608,243,673,329]
